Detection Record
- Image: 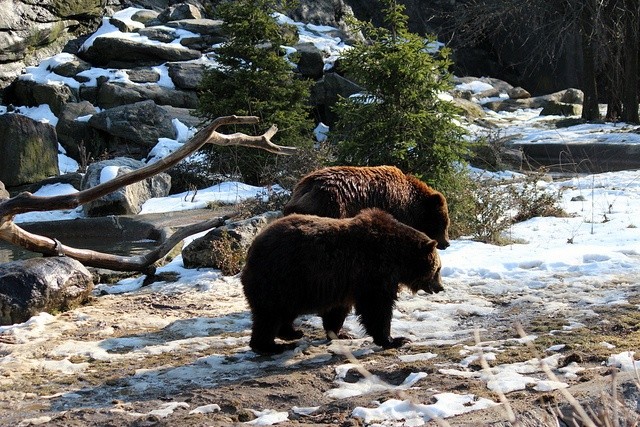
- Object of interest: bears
[239,206,445,357]
[283,164,451,251]
